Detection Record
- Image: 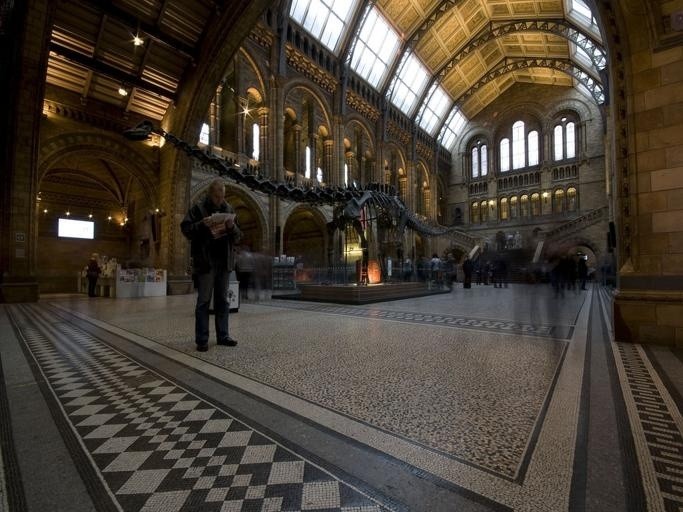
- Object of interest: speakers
[150,214,160,242]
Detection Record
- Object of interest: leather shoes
[197,344,209,352]
[217,337,238,347]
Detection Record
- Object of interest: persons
[397,244,591,300]
[86,253,102,298]
[178,178,246,353]
[234,244,272,304]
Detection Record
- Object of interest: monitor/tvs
[58,218,94,240]
